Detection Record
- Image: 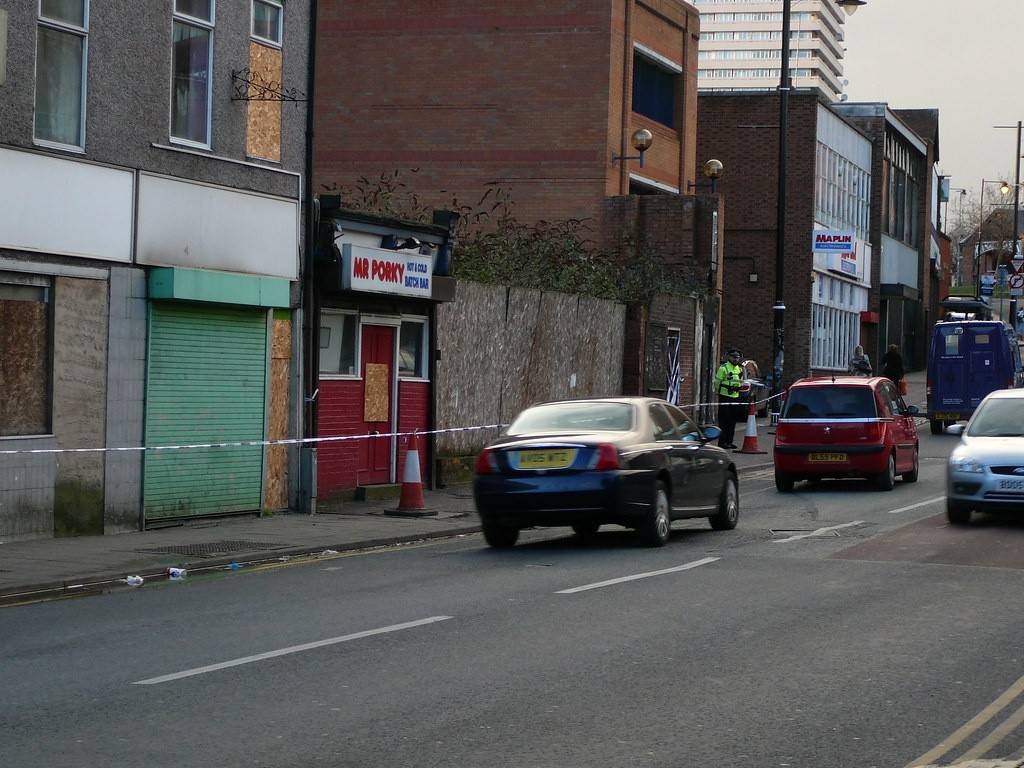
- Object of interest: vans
[925,319,1024,434]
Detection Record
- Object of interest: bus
[938,293,993,321]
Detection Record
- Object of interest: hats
[726,350,743,358]
[889,344,899,350]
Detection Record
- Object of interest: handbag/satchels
[857,355,871,375]
[898,378,908,396]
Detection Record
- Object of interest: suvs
[980,270,995,295]
[719,357,770,423]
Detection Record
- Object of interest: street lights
[943,187,967,235]
[977,178,1008,302]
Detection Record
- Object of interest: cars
[773,375,920,492]
[471,396,740,546]
[945,387,1024,525]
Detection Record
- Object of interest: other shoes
[730,444,737,449]
[718,442,730,449]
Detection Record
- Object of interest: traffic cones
[384,435,438,517]
[732,401,769,455]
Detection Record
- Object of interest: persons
[882,344,907,402]
[848,345,872,377]
[713,348,744,449]
